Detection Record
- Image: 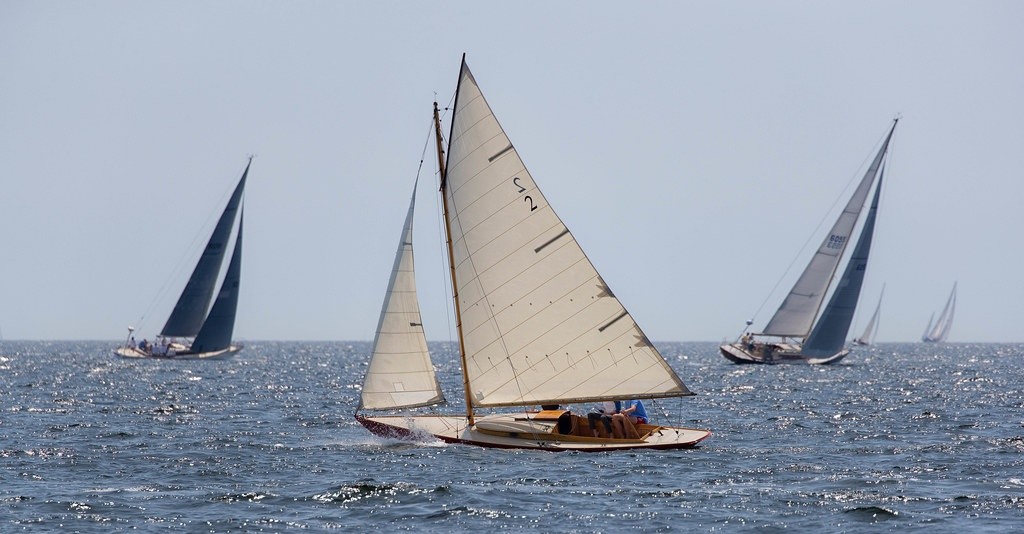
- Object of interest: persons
[741,333,755,351]
[613,399,648,439]
[139,339,146,350]
[131,337,136,350]
[587,400,622,438]
[152,337,173,358]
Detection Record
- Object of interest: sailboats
[851,284,886,347]
[353,53,714,452]
[922,281,958,344]
[719,117,898,366]
[112,154,253,360]
